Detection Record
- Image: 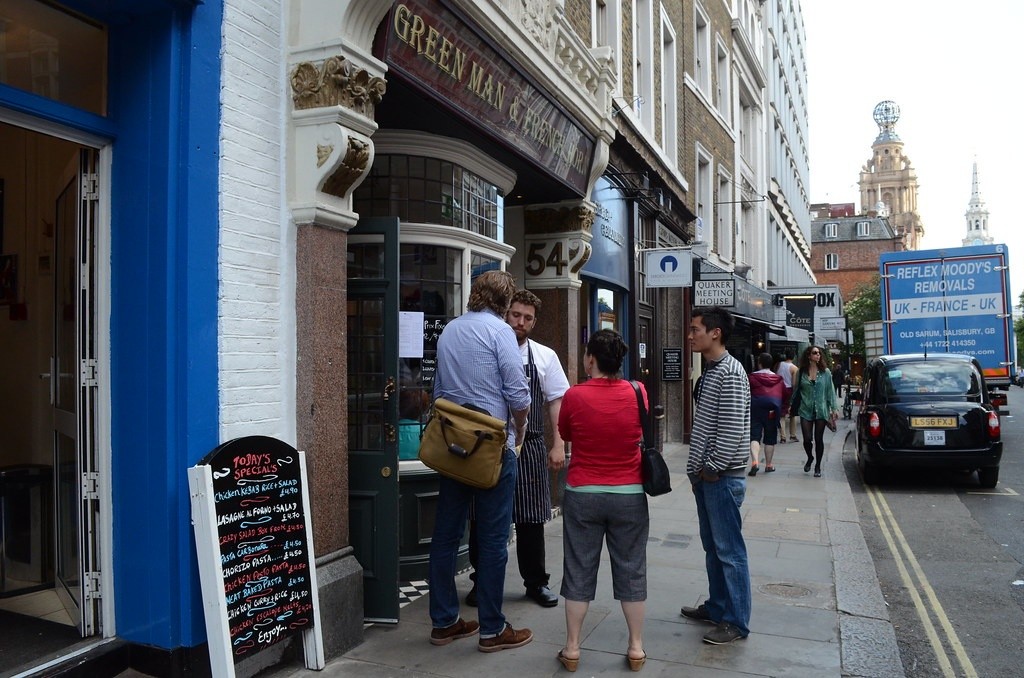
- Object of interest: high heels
[628,648,647,671]
[478,620,534,653]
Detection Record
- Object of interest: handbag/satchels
[790,366,804,416]
[419,397,510,490]
[643,446,672,497]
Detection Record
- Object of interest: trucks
[878,243,1016,425]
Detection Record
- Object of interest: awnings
[731,313,828,349]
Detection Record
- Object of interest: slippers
[557,647,580,672]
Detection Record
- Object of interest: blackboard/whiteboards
[196,434,321,665]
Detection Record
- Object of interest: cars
[1015,374,1021,386]
[847,353,1003,489]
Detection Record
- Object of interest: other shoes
[526,584,558,606]
[765,465,775,472]
[465,586,476,606]
[780,438,787,443]
[679,602,712,620]
[790,435,800,442]
[430,618,480,645]
[814,468,821,477]
[748,464,760,476]
[702,623,743,645]
[804,456,814,472]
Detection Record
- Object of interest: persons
[749,345,836,477]
[557,329,648,671]
[466,289,571,607]
[428,271,532,653]
[682,307,751,644]
[830,365,844,398]
